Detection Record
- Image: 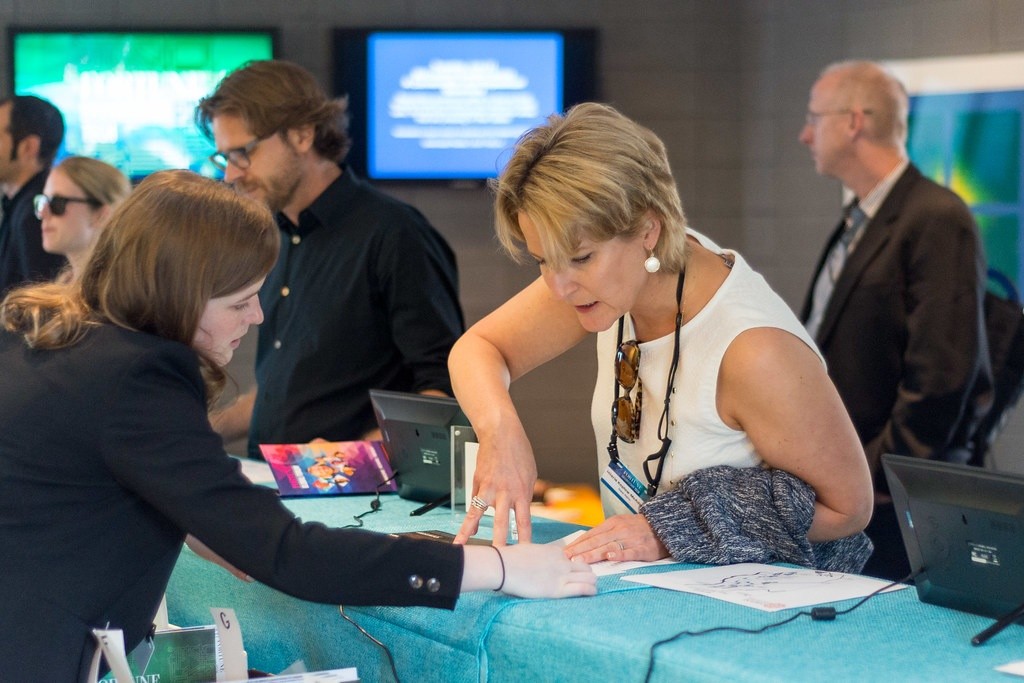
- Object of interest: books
[259,441,397,498]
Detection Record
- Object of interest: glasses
[611,340,642,444]
[805,109,874,127]
[209,136,263,171]
[33,194,96,220]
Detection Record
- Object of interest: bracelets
[490,545,505,591]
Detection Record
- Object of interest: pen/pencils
[971,606,1024,646]
[410,494,450,516]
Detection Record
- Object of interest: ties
[841,203,863,249]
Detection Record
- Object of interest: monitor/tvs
[330,26,598,191]
[4,23,283,188]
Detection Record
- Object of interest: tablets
[368,388,479,507]
[881,454,1024,626]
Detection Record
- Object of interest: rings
[473,496,488,511]
[615,541,624,550]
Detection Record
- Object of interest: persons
[449,102,873,564]
[0,96,63,296]
[196,61,468,465]
[0,171,599,683]
[798,61,993,580]
[34,158,130,276]
